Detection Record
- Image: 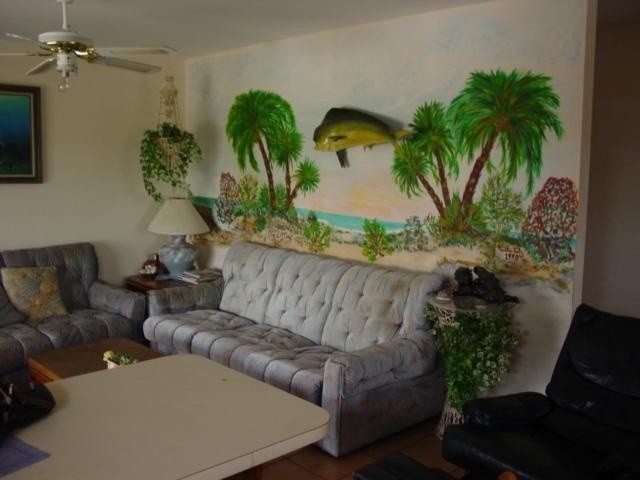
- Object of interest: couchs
[442,303,640,480]
[0,241,146,373]
[143,241,453,457]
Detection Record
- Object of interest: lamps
[56,49,78,92]
[146,198,210,277]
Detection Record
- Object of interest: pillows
[0,287,25,327]
[1,266,68,320]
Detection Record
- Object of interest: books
[177,269,221,284]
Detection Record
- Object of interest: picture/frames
[0,84,42,183]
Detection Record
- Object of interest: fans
[0,0,178,78]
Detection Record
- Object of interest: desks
[0,353,331,479]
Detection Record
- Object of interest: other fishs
[312,107,413,169]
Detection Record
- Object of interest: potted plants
[140,122,201,203]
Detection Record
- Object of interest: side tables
[426,290,518,441]
[124,273,193,293]
